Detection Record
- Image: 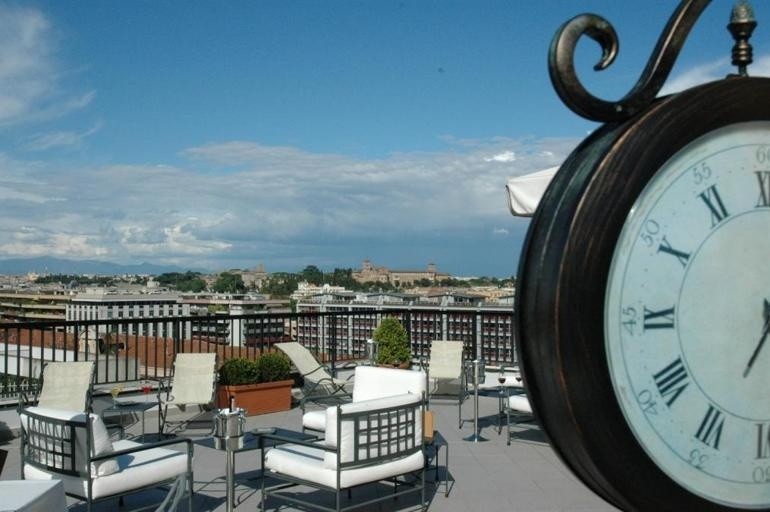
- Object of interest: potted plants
[373,318,412,369]
[219,353,294,417]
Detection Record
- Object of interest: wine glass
[497,376,506,394]
[110,386,121,410]
[514,371,523,391]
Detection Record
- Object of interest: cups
[142,384,151,404]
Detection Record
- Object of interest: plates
[251,427,277,435]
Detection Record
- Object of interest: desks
[463,385,524,445]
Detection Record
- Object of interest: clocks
[513,0,770,512]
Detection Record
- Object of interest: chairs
[20,352,215,510]
[507,393,533,417]
[274,340,358,400]
[258,363,454,512]
[429,339,464,397]
